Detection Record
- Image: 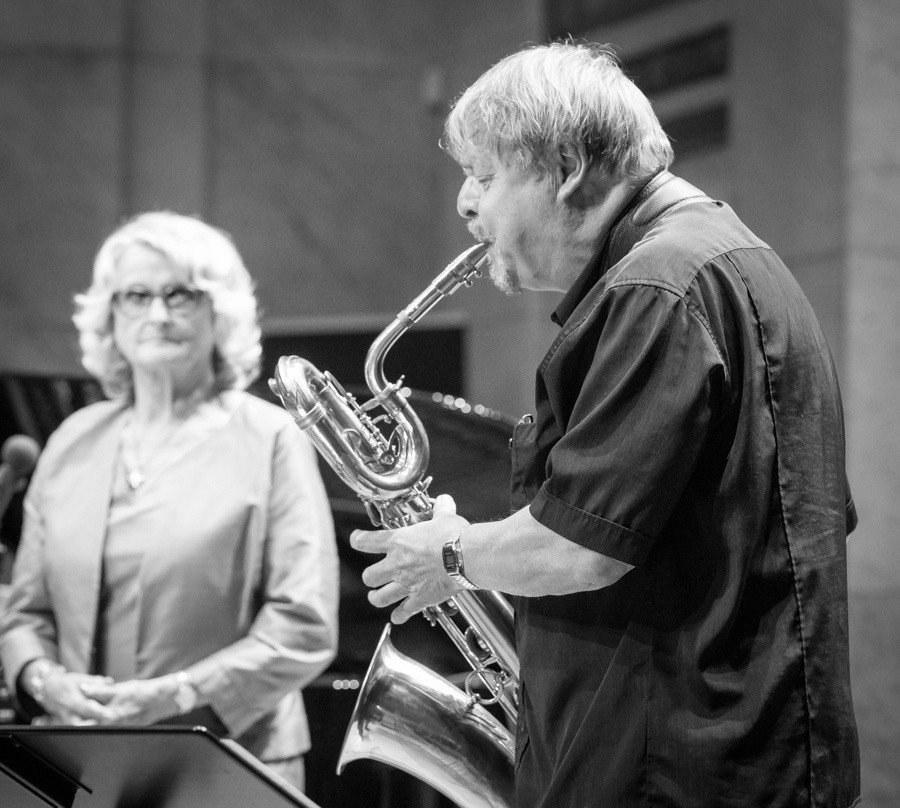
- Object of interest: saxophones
[261,242,522,808]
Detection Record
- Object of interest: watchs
[172,670,201,717]
[441,534,481,591]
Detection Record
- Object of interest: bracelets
[31,662,64,702]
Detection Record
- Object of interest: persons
[0,207,343,807]
[345,37,863,808]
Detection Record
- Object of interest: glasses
[114,288,204,319]
[518,414,533,425]
